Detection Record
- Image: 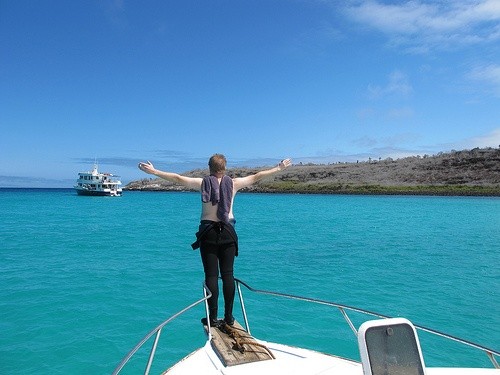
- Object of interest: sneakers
[202,316,218,327]
[225,311,235,325]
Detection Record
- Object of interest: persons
[138,153,293,328]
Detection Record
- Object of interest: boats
[73,156,122,197]
